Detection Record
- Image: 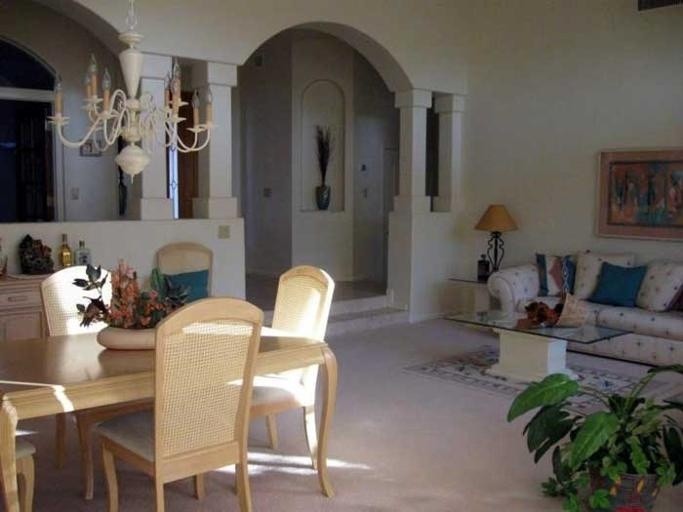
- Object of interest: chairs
[250,265,338,473]
[13,435,37,510]
[85,298,269,510]
[39,264,116,462]
[147,243,215,300]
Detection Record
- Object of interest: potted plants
[72,260,195,352]
[506,364,683,512]
[316,125,336,210]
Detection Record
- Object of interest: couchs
[488,250,683,365]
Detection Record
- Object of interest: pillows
[536,252,683,311]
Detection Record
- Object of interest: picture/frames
[595,148,683,242]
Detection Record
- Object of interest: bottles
[57,233,71,267]
[477,254,490,281]
[74,239,91,266]
[0,237,8,280]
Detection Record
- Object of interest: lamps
[50,0,216,179]
[474,204,519,272]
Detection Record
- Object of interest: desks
[0,330,337,511]
[441,282,488,319]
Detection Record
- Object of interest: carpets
[404,343,683,420]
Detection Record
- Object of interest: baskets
[553,293,589,327]
